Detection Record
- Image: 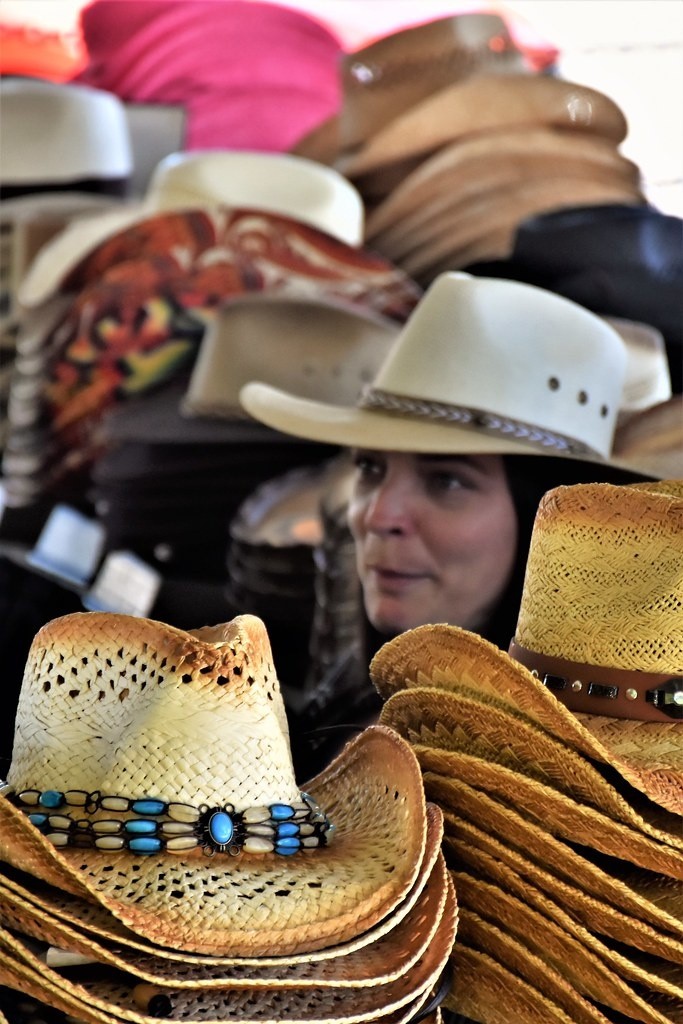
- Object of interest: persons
[346,273,624,654]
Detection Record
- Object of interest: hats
[0,0,683,482]
[238,271,664,484]
[0,486,683,1024]
